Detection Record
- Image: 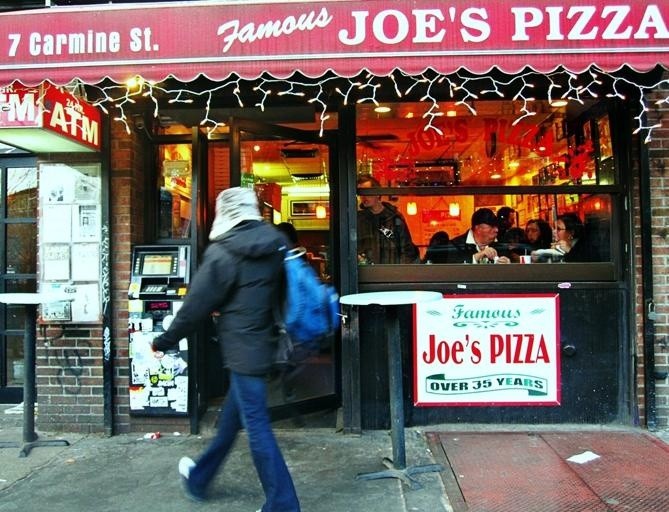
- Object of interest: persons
[424,207,603,262]
[356,176,421,263]
[151,187,301,512]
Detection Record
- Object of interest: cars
[520,254,532,265]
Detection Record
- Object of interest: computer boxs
[135,116,145,130]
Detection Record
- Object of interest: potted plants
[290,200,331,217]
[388,163,459,186]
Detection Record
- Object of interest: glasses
[448,196,461,218]
[315,158,327,219]
[406,194,418,216]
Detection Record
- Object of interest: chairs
[179,455,197,479]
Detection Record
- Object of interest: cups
[553,225,568,231]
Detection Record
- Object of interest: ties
[142,254,173,275]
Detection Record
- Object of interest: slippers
[277,244,340,344]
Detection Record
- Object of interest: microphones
[338,291,446,491]
[0,293,77,457]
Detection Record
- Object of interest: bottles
[472,209,498,225]
[209,187,261,240]
